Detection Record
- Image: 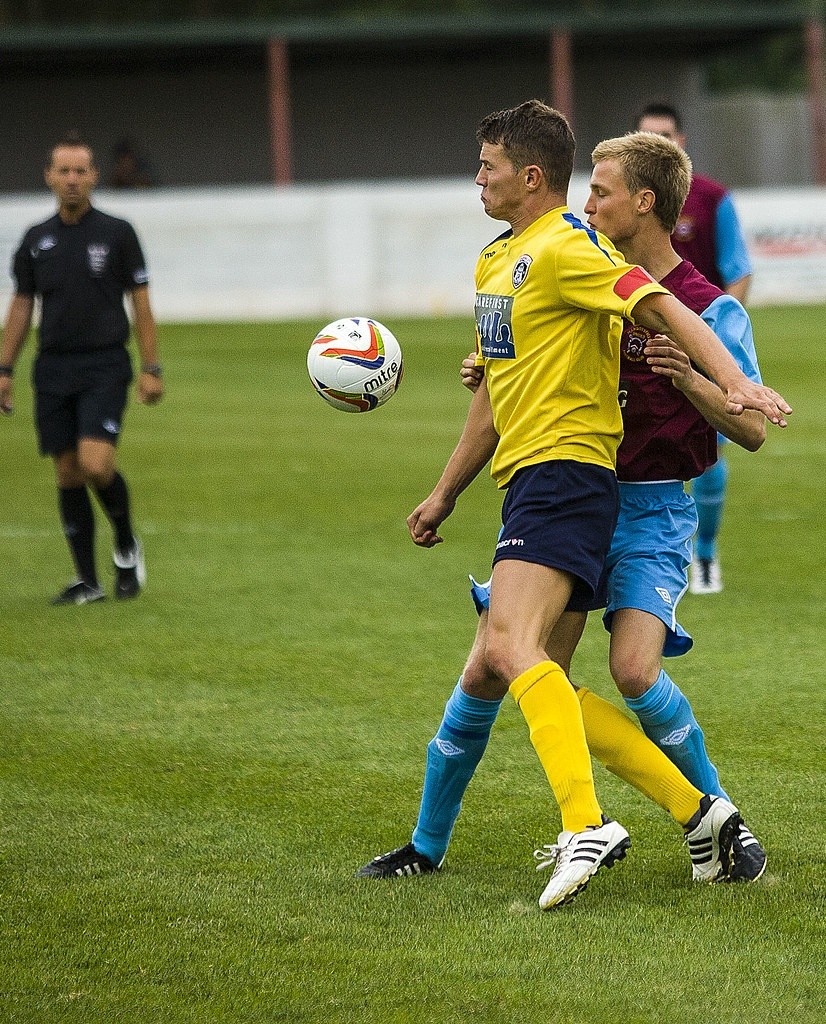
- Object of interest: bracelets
[143,364,161,378]
[0,366,13,376]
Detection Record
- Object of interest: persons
[109,140,153,188]
[357,132,767,882]
[0,139,164,606]
[405,100,794,912]
[634,102,752,594]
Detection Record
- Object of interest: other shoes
[691,553,724,596]
[112,531,143,600]
[50,579,106,609]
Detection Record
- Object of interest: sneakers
[681,791,746,884]
[726,822,767,883]
[355,842,445,881]
[533,814,631,913]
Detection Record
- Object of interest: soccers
[305,316,406,414]
